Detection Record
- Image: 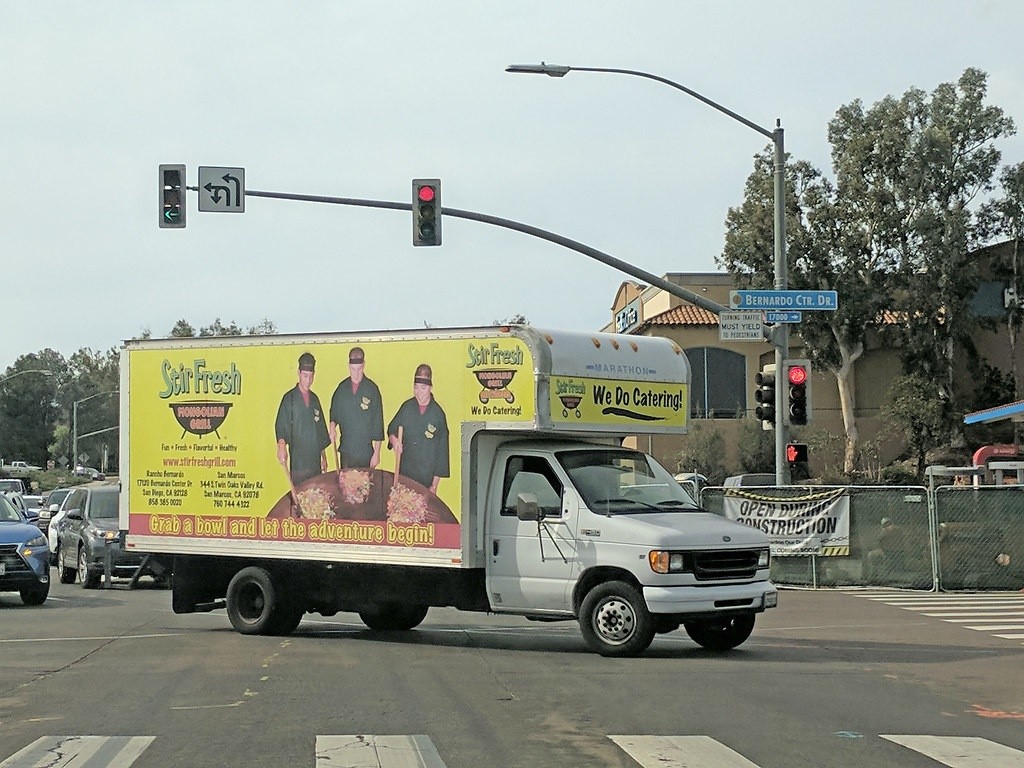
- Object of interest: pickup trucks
[2,460,44,479]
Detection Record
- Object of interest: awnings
[963,399,1024,425]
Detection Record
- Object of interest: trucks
[116,322,781,657]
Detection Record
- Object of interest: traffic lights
[785,442,809,463]
[157,162,187,232]
[754,364,776,431]
[779,357,814,427]
[411,178,443,247]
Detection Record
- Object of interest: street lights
[505,59,792,529]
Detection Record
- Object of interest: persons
[275,352,332,486]
[387,364,450,496]
[329,347,385,468]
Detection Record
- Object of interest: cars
[0,476,167,604]
[69,465,108,482]
